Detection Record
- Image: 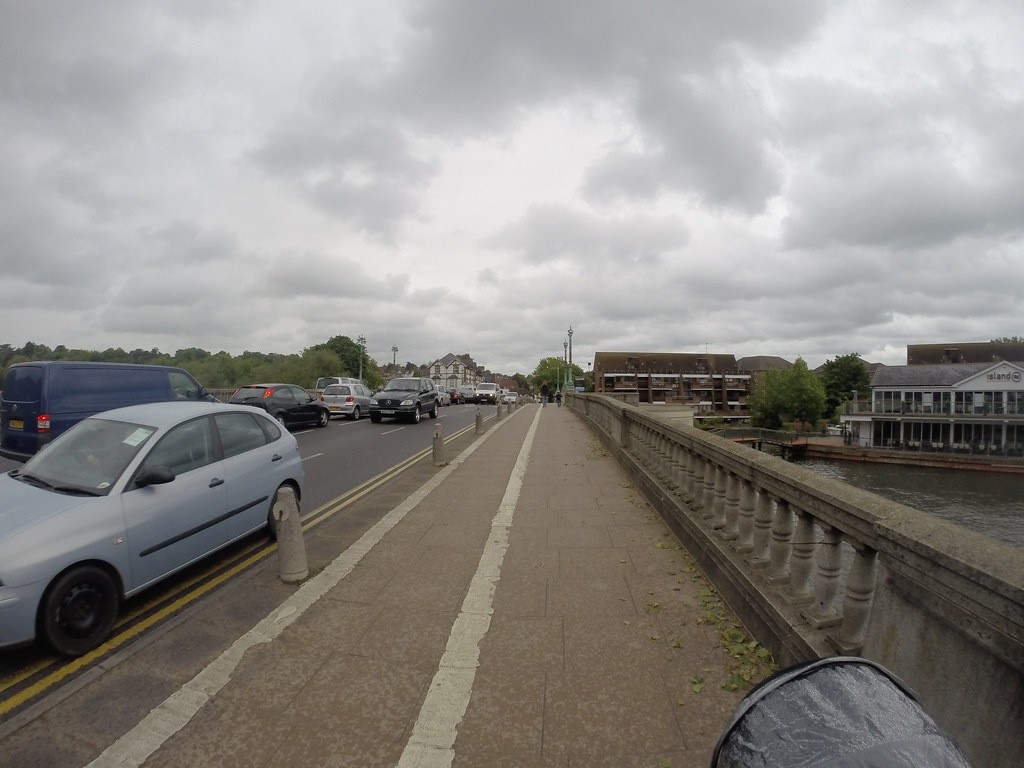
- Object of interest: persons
[555,392,563,406]
[547,394,554,403]
[540,382,548,408]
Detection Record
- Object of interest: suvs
[369,377,439,425]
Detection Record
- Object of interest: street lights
[568,325,574,385]
[356,337,367,380]
[391,346,398,375]
[562,340,568,389]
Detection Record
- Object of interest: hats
[557,391,560,392]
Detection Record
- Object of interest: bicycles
[555,396,562,408]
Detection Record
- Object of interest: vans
[475,383,500,406]
[0,360,223,466]
[315,377,362,399]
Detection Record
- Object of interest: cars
[502,391,519,404]
[226,383,331,430]
[318,384,374,420]
[0,401,303,662]
[433,385,452,408]
[447,388,465,405]
